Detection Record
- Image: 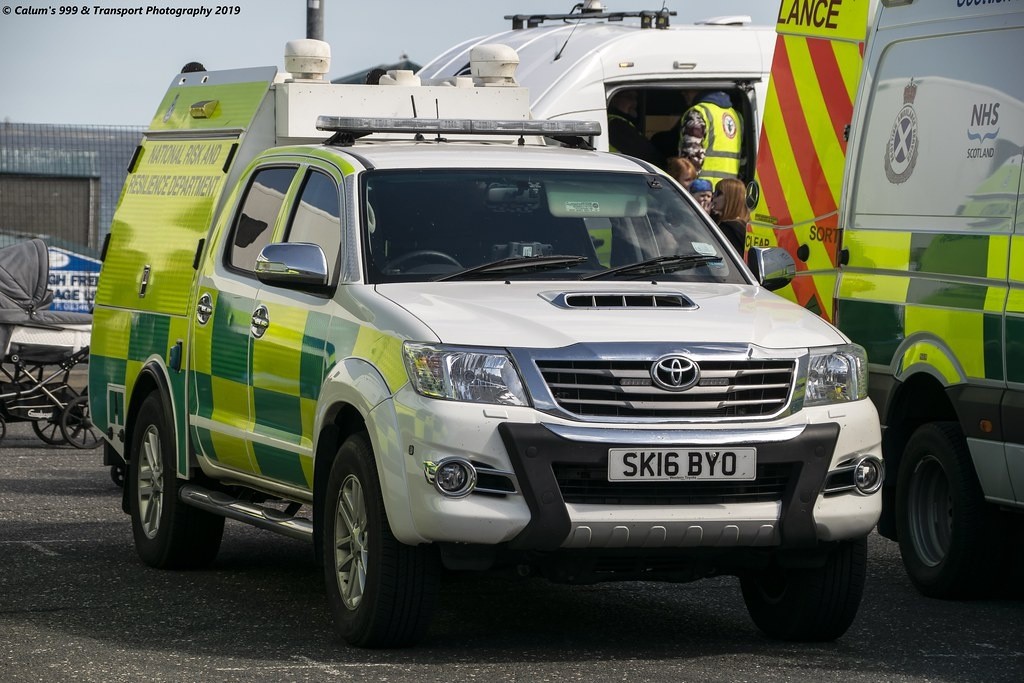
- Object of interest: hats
[688,179,712,194]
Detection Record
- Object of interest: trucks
[87,36,889,650]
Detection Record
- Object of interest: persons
[679,89,743,190]
[667,157,696,188]
[608,91,666,171]
[700,178,749,259]
[689,179,713,207]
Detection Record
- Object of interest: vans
[836,1,1024,601]
[406,0,780,192]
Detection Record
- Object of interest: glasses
[714,190,723,197]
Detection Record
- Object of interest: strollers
[0,238,107,451]
